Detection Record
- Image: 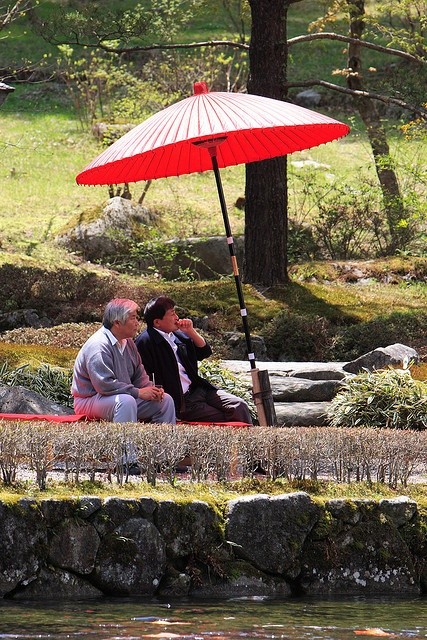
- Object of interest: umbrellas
[76,82,350,370]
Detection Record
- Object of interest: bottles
[151,385,164,402]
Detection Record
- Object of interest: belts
[184,384,196,398]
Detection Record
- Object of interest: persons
[133,296,254,426]
[71,298,176,425]
